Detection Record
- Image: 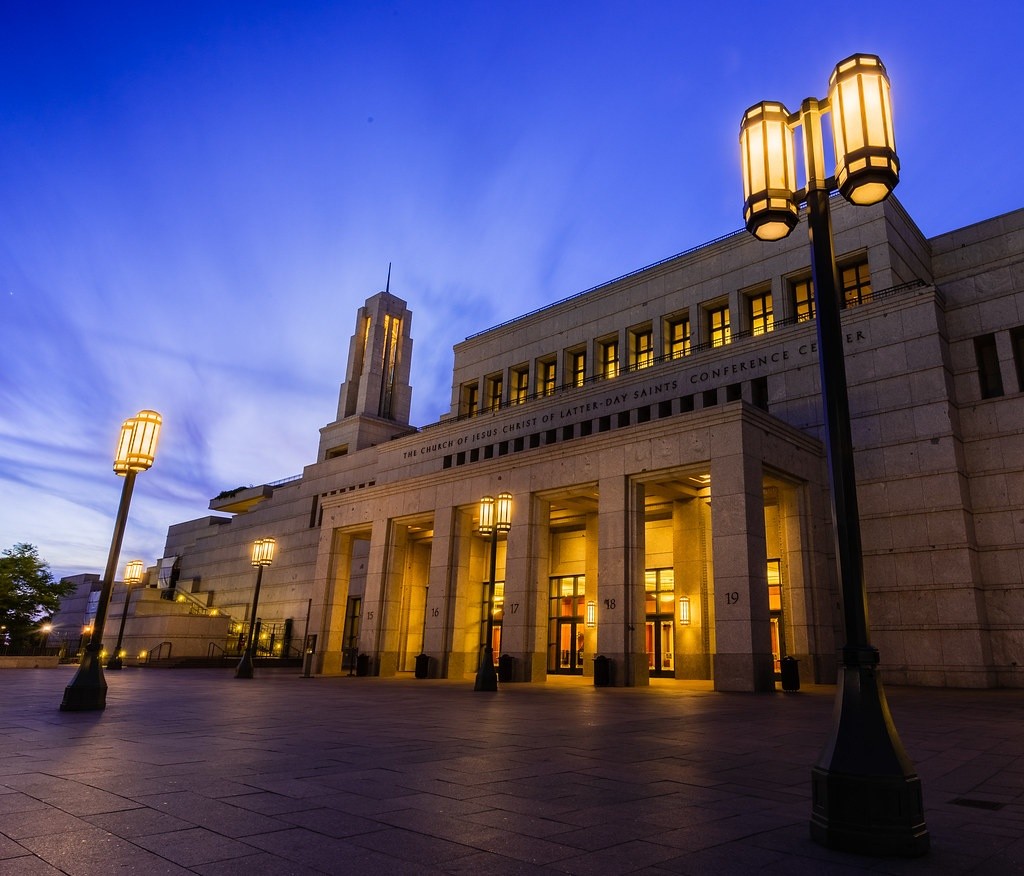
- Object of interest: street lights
[105,558,145,671]
[729,51,935,856]
[231,536,278,678]
[55,408,166,710]
[471,490,513,693]
[38,624,54,646]
[77,624,91,664]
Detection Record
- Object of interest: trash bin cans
[780,656,800,693]
[496,654,515,683]
[592,654,614,687]
[356,653,371,676]
[414,654,432,679]
